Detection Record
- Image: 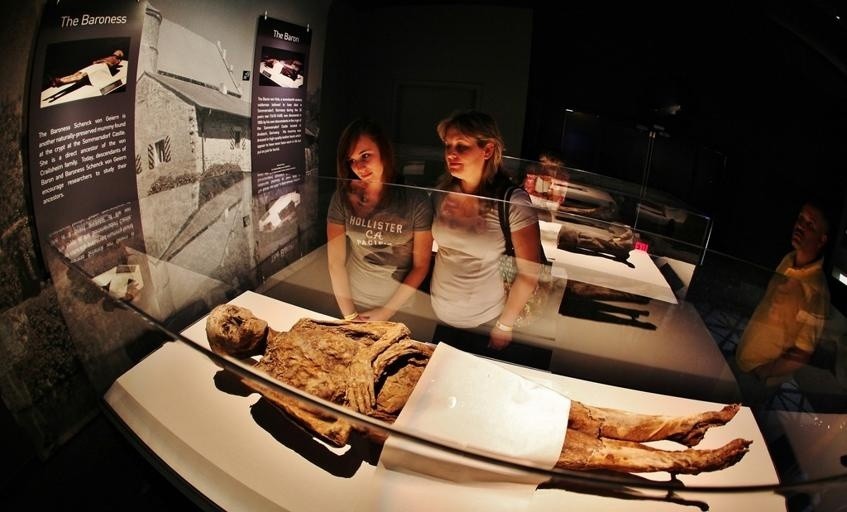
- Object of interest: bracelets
[495,321,515,332]
[343,310,357,322]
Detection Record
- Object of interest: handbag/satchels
[496,183,553,318]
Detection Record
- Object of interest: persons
[558,227,636,261]
[430,111,542,350]
[557,204,617,230]
[326,118,435,324]
[262,56,300,77]
[207,305,754,475]
[560,283,650,319]
[729,199,832,422]
[47,50,124,87]
